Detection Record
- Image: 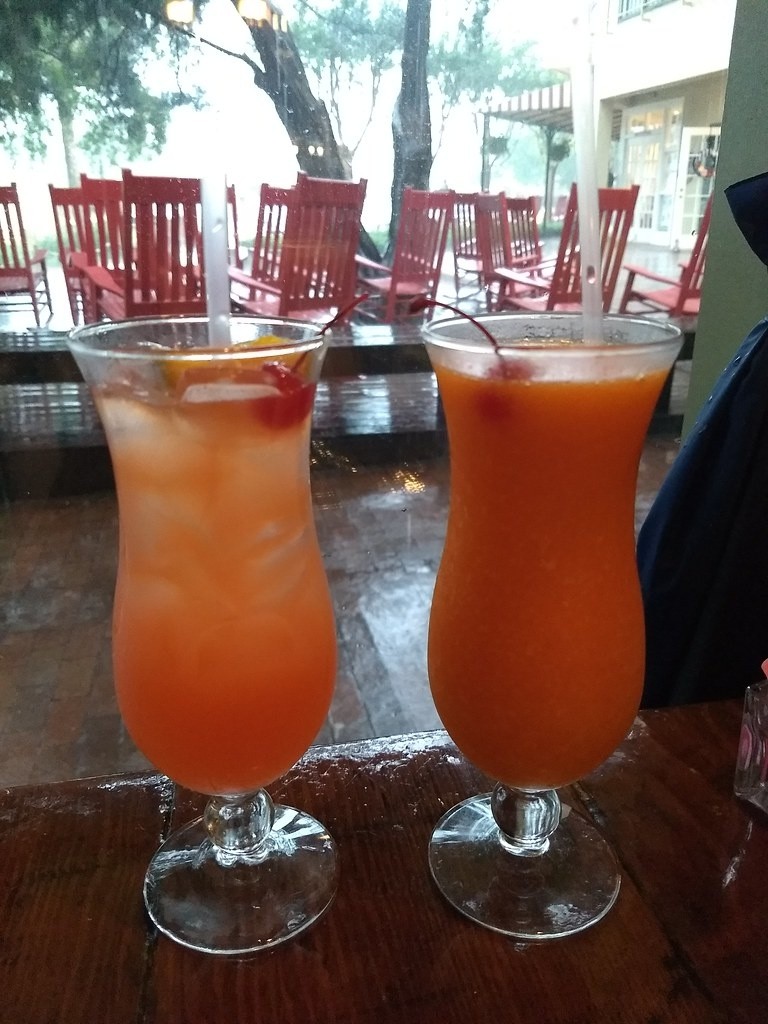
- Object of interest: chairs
[0,168,716,332]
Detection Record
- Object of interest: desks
[1,693,768,1024]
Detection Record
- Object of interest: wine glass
[418,311,688,941]
[66,314,345,958]
